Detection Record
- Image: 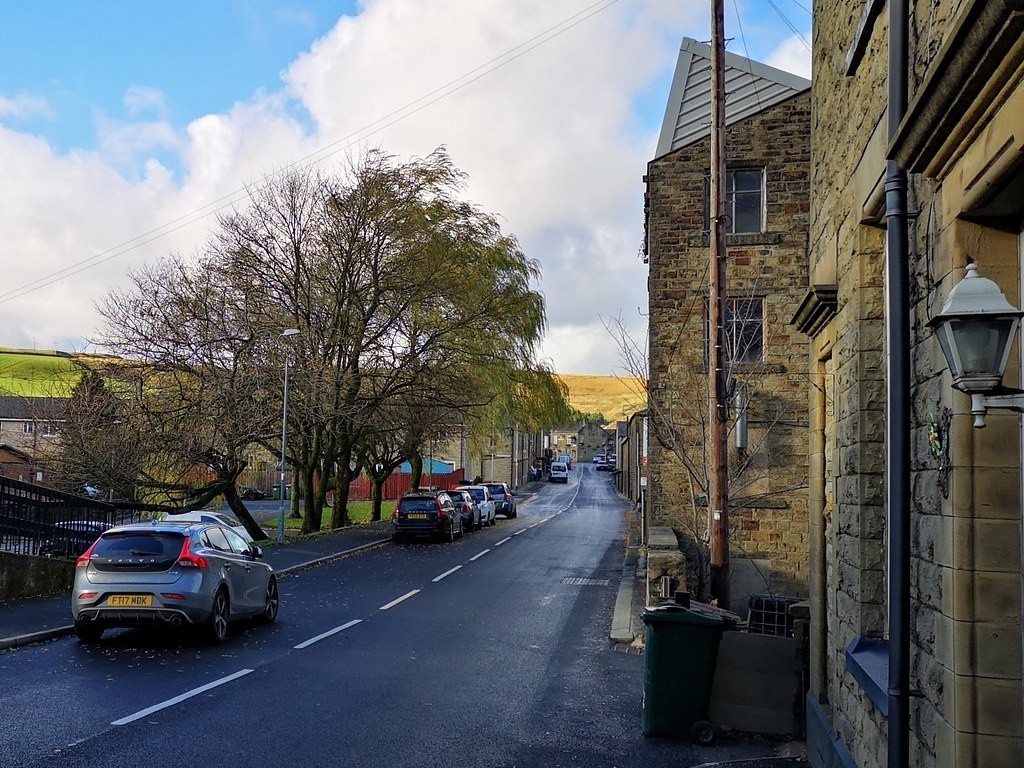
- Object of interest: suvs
[391,489,465,542]
[71,522,281,643]
[477,482,517,518]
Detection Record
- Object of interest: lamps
[923,254,1024,429]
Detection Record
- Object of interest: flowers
[924,409,953,499]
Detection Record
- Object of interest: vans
[560,455,573,470]
[550,462,569,483]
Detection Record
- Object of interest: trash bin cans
[638,602,736,747]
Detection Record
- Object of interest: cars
[40,520,120,558]
[455,486,497,526]
[592,453,608,470]
[159,513,270,545]
[439,491,482,532]
[191,482,266,501]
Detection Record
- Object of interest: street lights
[276,328,300,547]
